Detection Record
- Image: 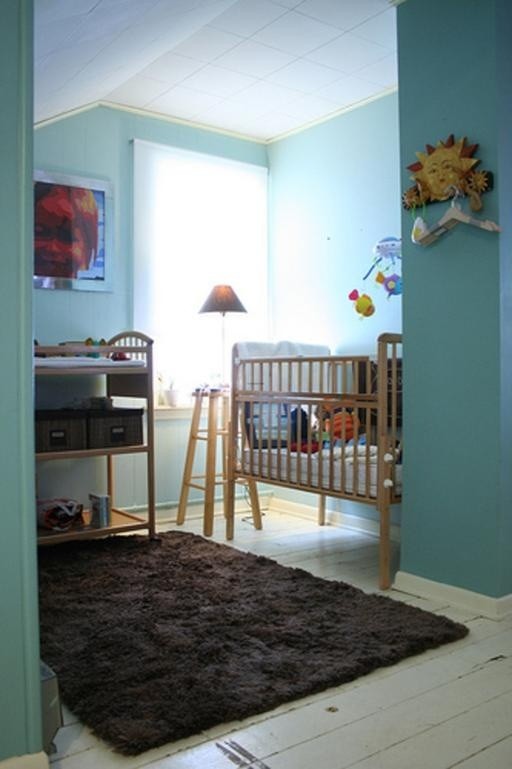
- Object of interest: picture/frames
[33,170,117,294]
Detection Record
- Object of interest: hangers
[417,185,502,248]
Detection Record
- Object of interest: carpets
[38,529,470,759]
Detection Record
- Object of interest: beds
[226,332,403,589]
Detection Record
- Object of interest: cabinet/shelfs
[32,329,156,549]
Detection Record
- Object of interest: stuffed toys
[324,405,361,441]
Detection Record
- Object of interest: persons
[35,181,98,278]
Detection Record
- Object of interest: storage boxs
[35,407,144,453]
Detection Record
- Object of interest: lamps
[198,284,249,386]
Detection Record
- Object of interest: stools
[177,390,263,538]
